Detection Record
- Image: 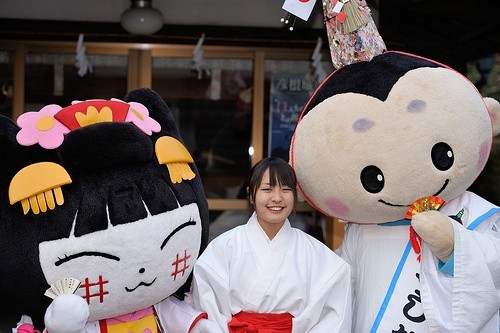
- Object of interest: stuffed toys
[0,87,224,333]
[280,0,500,333]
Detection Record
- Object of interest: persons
[193,157,352,333]
